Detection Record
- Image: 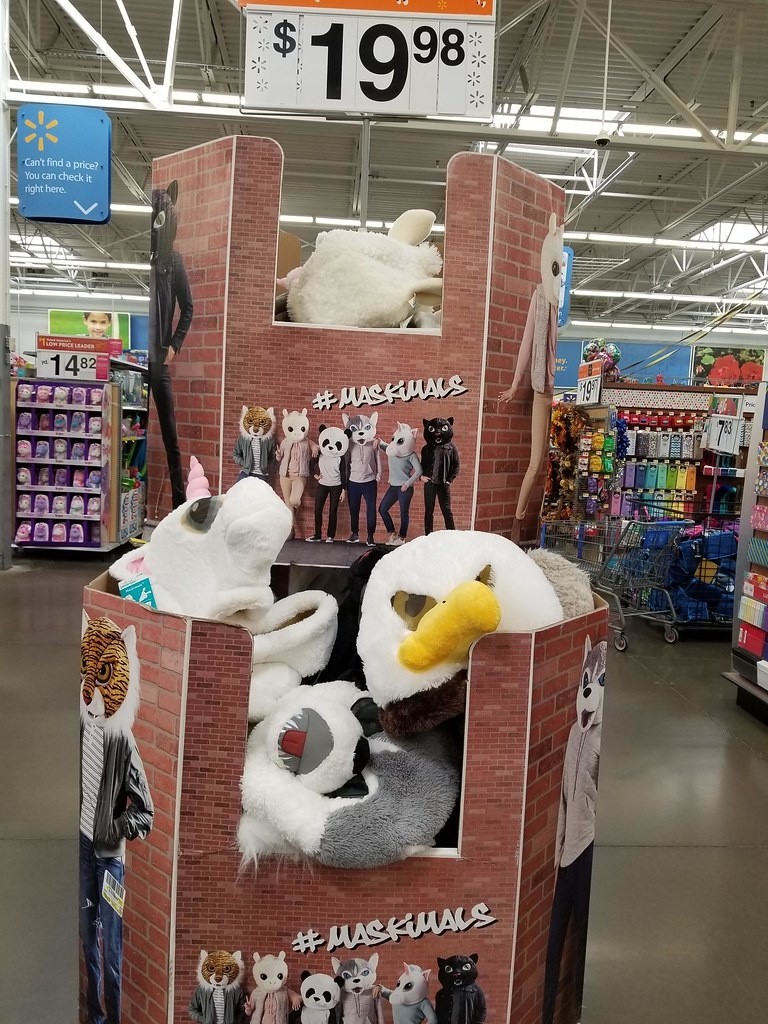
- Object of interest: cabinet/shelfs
[11,357,149,552]
[720,382,768,728]
[575,384,758,609]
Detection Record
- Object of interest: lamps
[0,0,768,336]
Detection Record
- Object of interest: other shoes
[366,537,376,547]
[391,536,405,546]
[286,532,295,541]
[386,533,398,546]
[305,536,321,542]
[346,534,359,543]
[326,537,333,543]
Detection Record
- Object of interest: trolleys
[540,516,696,652]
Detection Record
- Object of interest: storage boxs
[737,622,766,657]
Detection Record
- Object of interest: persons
[186,949,487,1024]
[80,312,112,340]
[497,214,564,545]
[543,633,608,1024]
[148,180,194,511]
[233,405,460,546]
[79,610,153,1024]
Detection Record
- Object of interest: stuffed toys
[106,477,594,869]
[278,208,445,328]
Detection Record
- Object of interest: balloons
[584,338,622,382]
